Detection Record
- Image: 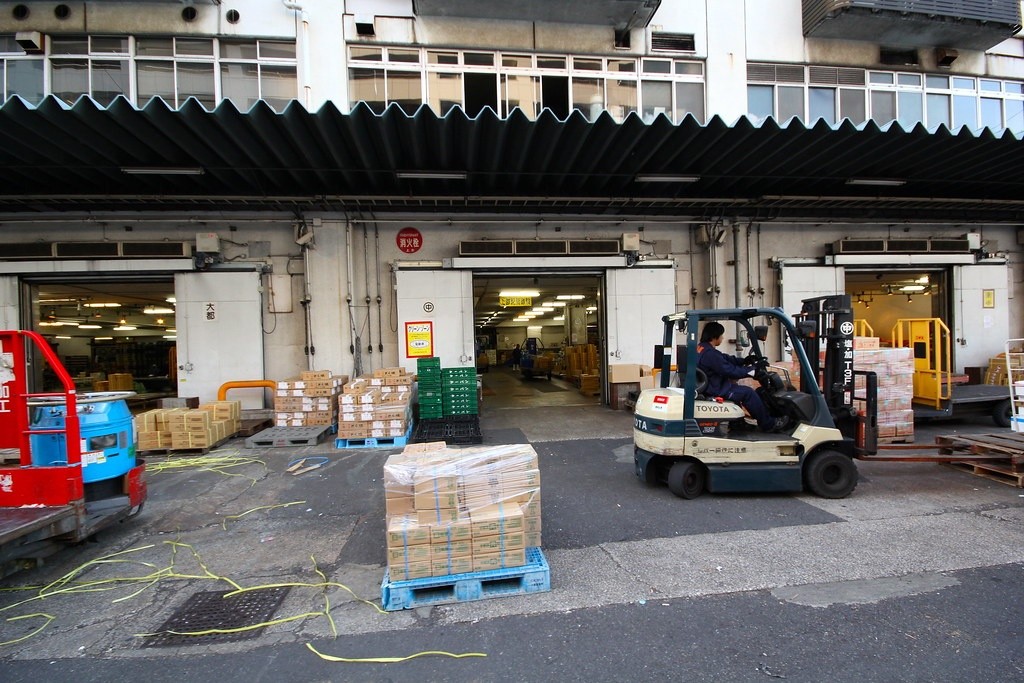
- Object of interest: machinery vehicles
[632,293,1012,499]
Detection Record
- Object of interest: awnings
[2,91,1024,226]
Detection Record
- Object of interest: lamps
[887,284,894,296]
[865,302,870,309]
[907,295,912,303]
[857,295,862,304]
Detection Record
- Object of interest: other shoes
[768,416,788,432]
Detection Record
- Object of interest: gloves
[755,360,770,369]
[745,355,757,364]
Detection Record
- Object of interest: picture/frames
[983,288,995,309]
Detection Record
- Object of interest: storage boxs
[964,366,988,385]
[383,440,543,581]
[134,399,241,449]
[738,334,915,437]
[607,363,655,410]
[338,367,417,438]
[273,370,349,426]
[983,345,1024,386]
[479,343,600,375]
[581,375,600,393]
[417,356,479,419]
[1011,381,1024,433]
[93,372,134,391]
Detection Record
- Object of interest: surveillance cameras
[715,231,727,244]
[296,232,313,246]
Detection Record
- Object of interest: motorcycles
[520,337,552,381]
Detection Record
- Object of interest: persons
[512,344,521,371]
[697,322,789,433]
[477,340,480,357]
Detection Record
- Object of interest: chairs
[677,343,707,399]
